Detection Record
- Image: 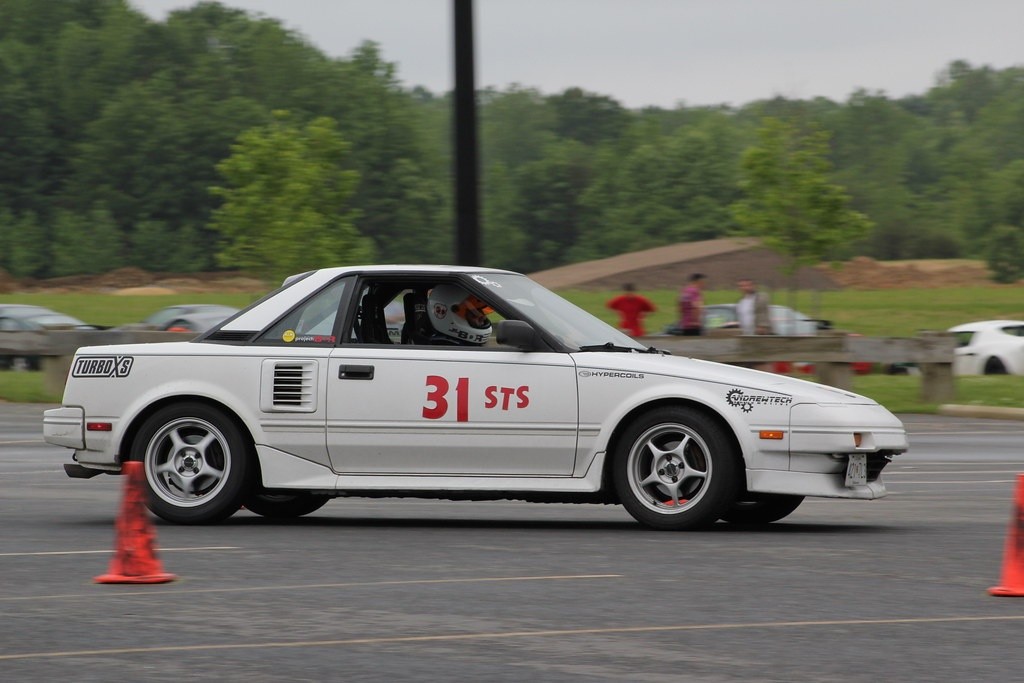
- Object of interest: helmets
[426,283,496,344]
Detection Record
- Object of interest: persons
[428,285,495,348]
[607,283,657,339]
[735,279,774,373]
[680,273,708,337]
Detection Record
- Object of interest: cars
[42,262,908,533]
[909,319,1024,383]
[142,303,244,335]
[0,303,100,373]
[645,302,872,377]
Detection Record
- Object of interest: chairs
[362,294,393,345]
[401,292,435,344]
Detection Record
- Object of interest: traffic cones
[985,473,1024,597]
[93,461,178,584]
